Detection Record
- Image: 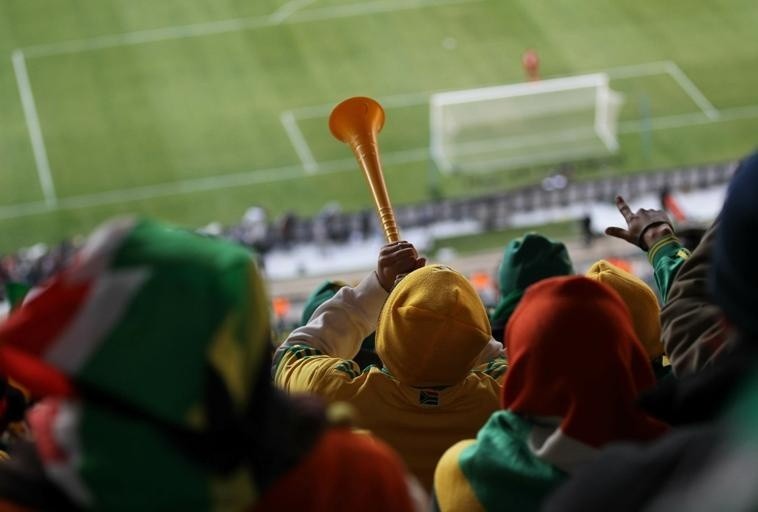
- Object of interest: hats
[715,153,758,337]
[589,259,661,362]
[376,266,491,388]
[301,281,354,326]
[498,234,574,294]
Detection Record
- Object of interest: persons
[0,155,758,512]
[517,47,541,82]
